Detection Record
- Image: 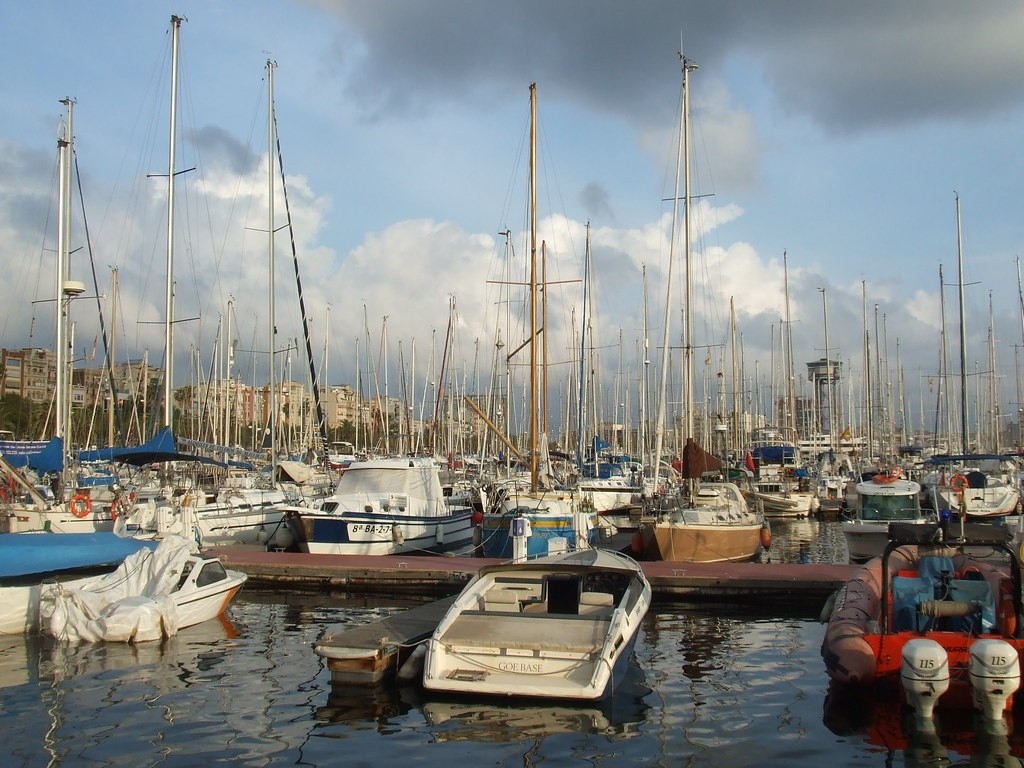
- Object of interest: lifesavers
[111,499,123,522]
[70,494,91,518]
[0,482,11,504]
[950,475,968,492]
[893,467,901,477]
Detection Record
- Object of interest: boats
[34,534,248,643]
[420,547,653,706]
[816,535,1023,733]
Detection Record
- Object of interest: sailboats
[1,14,1024,576]
